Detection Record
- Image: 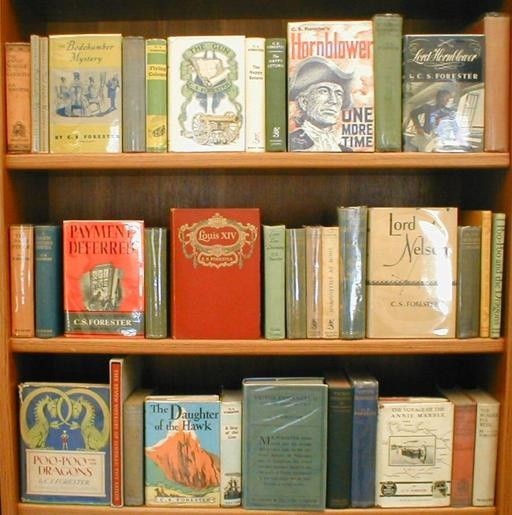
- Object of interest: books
[10,207,505,339]
[5,34,287,153]
[287,20,375,153]
[371,14,512,153]
[16,355,502,511]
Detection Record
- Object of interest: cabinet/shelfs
[0,0,512,515]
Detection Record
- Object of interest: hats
[290,59,352,109]
